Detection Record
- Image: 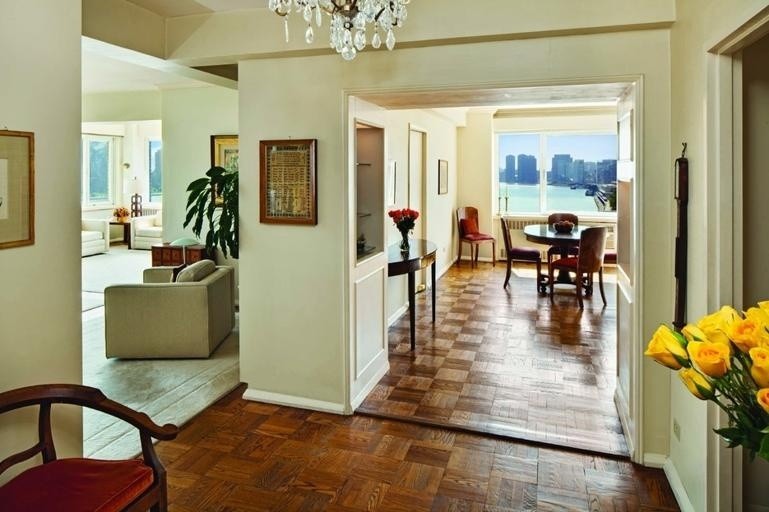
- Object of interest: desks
[385,236,444,356]
[109,220,129,245]
[520,221,610,291]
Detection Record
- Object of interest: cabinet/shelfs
[352,116,387,268]
[151,242,210,265]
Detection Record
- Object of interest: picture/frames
[255,136,322,229]
[207,131,240,212]
[436,157,450,197]
[0,127,39,252]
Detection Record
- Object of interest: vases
[116,216,127,225]
[398,238,414,262]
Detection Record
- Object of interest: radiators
[502,216,557,262]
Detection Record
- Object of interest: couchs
[128,210,166,251]
[102,258,236,363]
[80,213,111,260]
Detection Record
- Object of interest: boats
[569,186,577,189]
[583,188,595,196]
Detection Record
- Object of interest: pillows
[169,263,184,284]
[461,217,479,235]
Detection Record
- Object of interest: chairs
[549,226,613,310]
[0,376,184,511]
[451,204,501,270]
[545,211,582,232]
[499,216,543,297]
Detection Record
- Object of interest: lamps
[263,0,418,66]
[170,237,199,264]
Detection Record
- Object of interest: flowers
[386,207,421,233]
[635,290,769,467]
[110,207,131,218]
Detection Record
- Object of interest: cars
[600,184,615,192]
